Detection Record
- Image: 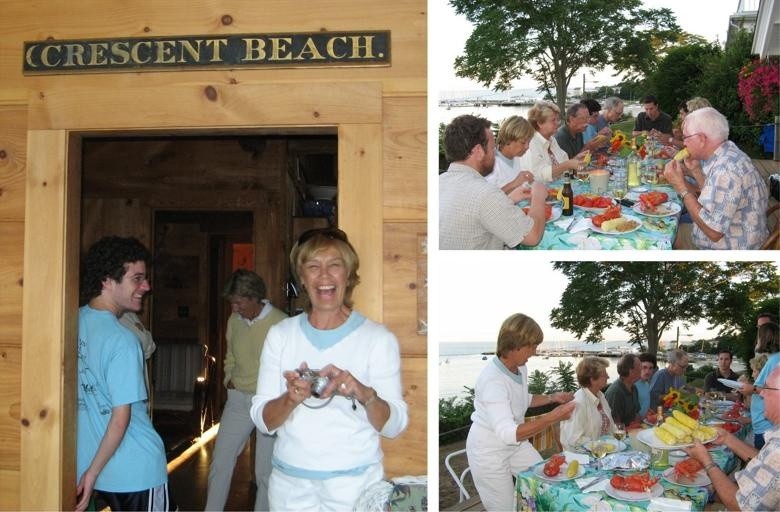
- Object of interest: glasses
[679,132,707,142]
[298,226,355,252]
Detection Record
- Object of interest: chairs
[445,448,471,503]
[759,174,780,250]
[147,341,218,437]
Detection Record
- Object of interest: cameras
[299,369,336,398]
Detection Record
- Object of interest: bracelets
[549,391,553,403]
[363,392,378,406]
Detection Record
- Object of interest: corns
[566,459,578,478]
[672,148,690,162]
[601,216,626,232]
[583,153,591,166]
[655,408,717,446]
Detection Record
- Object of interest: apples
[543,453,565,476]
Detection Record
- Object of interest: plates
[512,134,684,236]
[536,378,744,502]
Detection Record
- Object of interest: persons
[558,313,780,511]
[204,268,288,511]
[249,227,409,510]
[74,235,180,511]
[119,310,156,420]
[440,94,769,249]
[465,313,574,512]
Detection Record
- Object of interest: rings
[293,386,301,394]
[339,382,346,389]
